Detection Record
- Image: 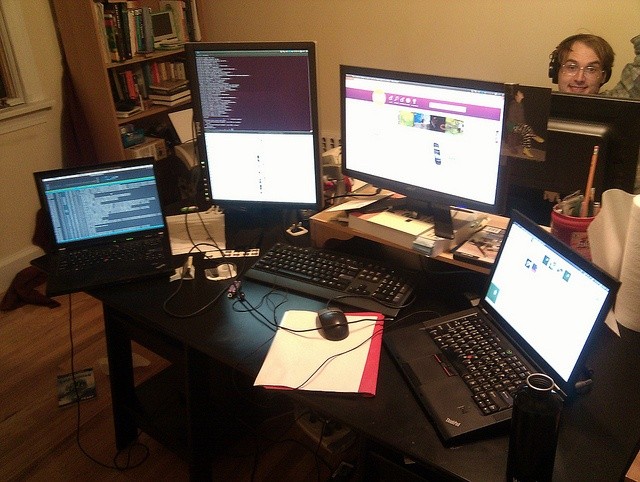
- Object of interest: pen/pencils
[590,187,600,216]
[471,238,488,259]
[580,145,599,218]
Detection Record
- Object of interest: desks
[30,184,637,481]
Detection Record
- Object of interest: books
[348,193,490,256]
[56,367,96,409]
[453,224,508,269]
[95,0,193,118]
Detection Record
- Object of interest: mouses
[317,306,348,340]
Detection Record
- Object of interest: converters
[432,295,474,315]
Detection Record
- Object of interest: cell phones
[454,225,507,268]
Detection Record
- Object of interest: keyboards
[244,241,416,320]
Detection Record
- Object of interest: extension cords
[203,245,260,261]
[295,406,357,454]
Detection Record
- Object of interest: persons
[505,89,545,158]
[557,34,614,94]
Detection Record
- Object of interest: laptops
[31,157,173,299]
[383,208,622,447]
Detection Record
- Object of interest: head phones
[601,68,613,87]
[548,62,561,85]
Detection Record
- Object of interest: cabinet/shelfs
[51,1,200,163]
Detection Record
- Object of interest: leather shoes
[535,136,544,143]
[523,149,535,158]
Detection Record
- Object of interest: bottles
[505,373,561,481]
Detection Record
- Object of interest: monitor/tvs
[184,42,325,206]
[496,83,640,223]
[339,65,508,240]
[150,11,178,42]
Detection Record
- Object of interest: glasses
[561,63,604,77]
